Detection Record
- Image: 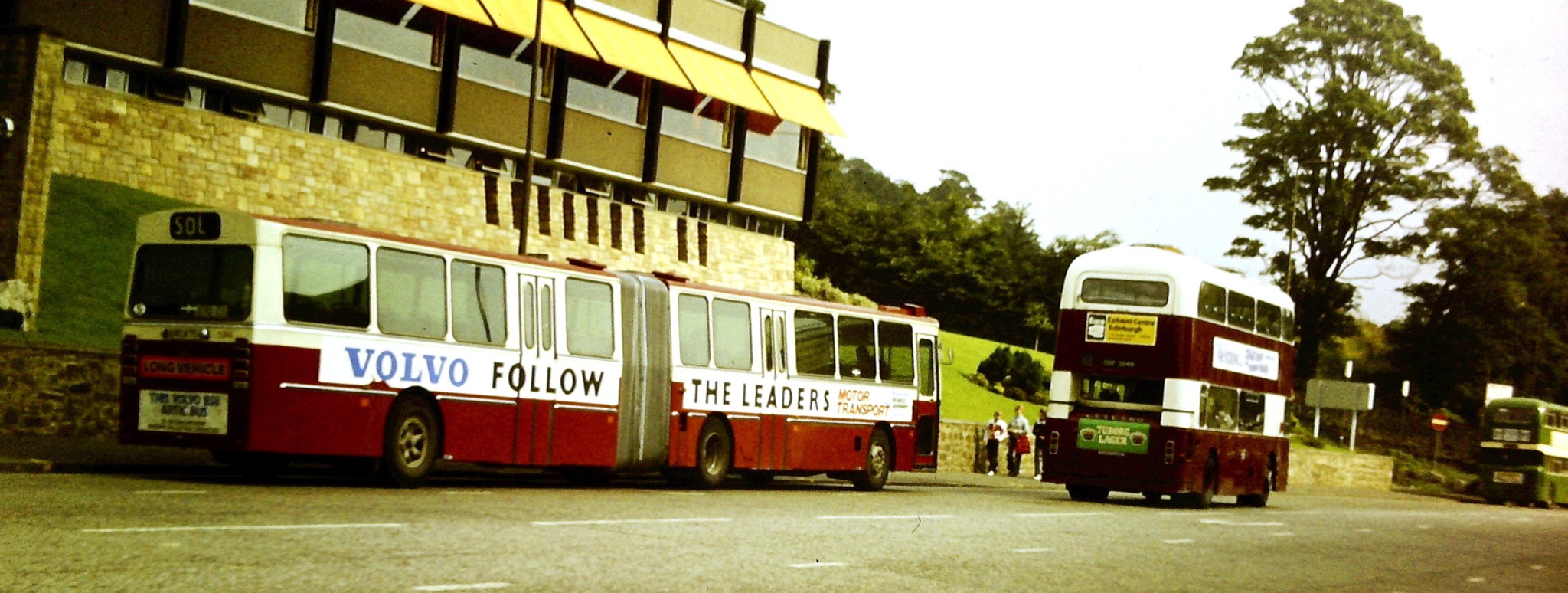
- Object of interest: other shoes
[1007,471,1018,477]
[1033,474,1043,480]
[987,470,995,476]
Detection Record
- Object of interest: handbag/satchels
[1015,430,1031,454]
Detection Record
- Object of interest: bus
[117,206,955,490]
[1478,397,1568,508]
[1040,245,1296,510]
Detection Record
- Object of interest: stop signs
[1431,414,1450,432]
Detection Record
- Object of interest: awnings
[414,0,848,140]
[324,0,784,136]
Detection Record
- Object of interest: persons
[1209,399,1239,431]
[1006,406,1031,477]
[848,346,891,380]
[983,412,1009,476]
[1032,408,1048,481]
